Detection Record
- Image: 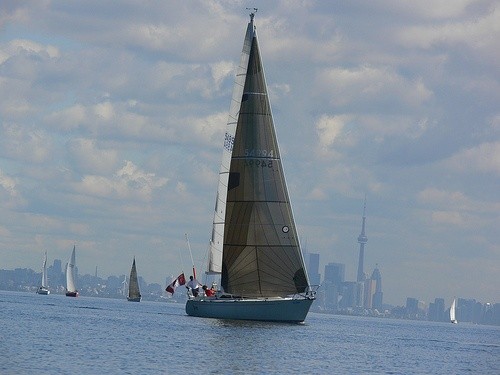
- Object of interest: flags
[166,273,186,296]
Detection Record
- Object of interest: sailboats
[65,240,78,297]
[126,255,142,302]
[449,298,458,324]
[184,7,320,323]
[37,250,51,295]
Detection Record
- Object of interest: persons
[186,276,203,297]
[202,285,214,298]
